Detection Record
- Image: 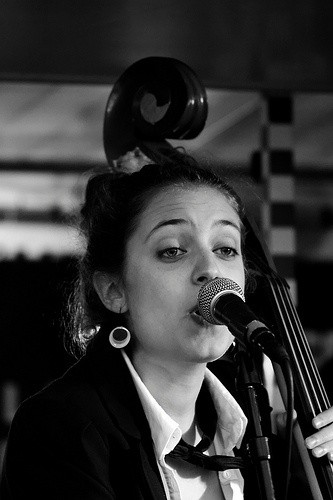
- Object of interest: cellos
[101,54,333,500]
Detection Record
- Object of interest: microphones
[195,276,290,364]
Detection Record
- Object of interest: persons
[0,142,333,500]
